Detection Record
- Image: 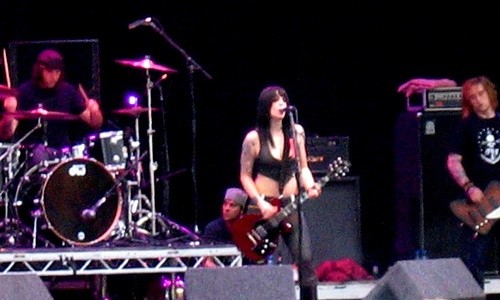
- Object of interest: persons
[199,86,322,300]
[0,49,104,164]
[446,76,500,294]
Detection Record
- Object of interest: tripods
[103,21,233,247]
[0,115,55,246]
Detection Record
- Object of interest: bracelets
[463,180,474,194]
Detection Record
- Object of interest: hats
[224,188,248,208]
[37,49,64,66]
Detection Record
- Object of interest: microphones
[282,106,294,112]
[81,209,96,221]
[128,17,152,29]
[153,74,168,87]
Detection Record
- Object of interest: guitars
[231,157,350,261]
[450,180,500,235]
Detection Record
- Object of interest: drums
[60,143,88,162]
[32,156,123,247]
[85,129,128,170]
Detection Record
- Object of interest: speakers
[365,257,486,300]
[397,112,500,276]
[289,174,365,268]
[0,273,55,300]
[183,265,296,300]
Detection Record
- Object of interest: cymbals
[1,108,81,120]
[113,107,159,114]
[0,84,20,99]
[116,58,177,73]
